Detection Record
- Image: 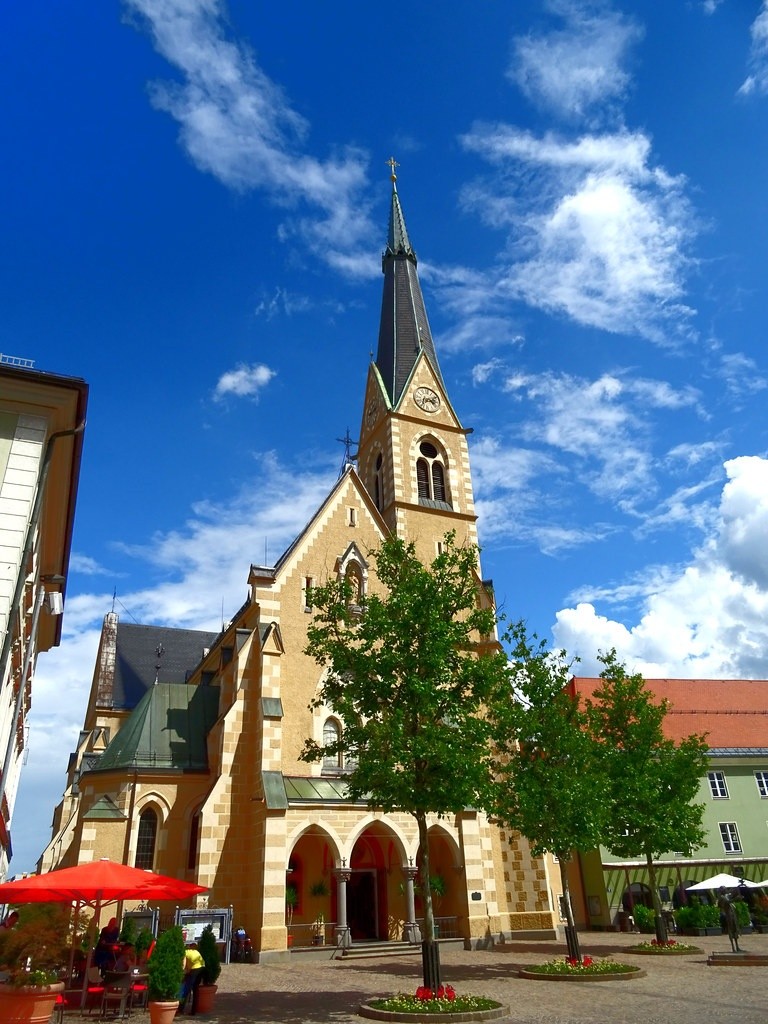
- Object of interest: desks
[104,974,149,1018]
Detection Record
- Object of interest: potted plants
[704,904,723,936]
[308,880,330,947]
[673,906,695,936]
[196,924,221,1014]
[633,904,655,934]
[421,876,448,939]
[690,895,706,936]
[753,904,768,934]
[0,902,90,1024]
[286,880,299,949]
[146,925,186,1024]
[732,901,753,935]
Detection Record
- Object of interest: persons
[0,912,20,934]
[175,946,206,1017]
[93,918,120,975]
[718,886,744,953]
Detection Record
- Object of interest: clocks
[366,399,379,427]
[413,387,441,413]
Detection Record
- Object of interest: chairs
[86,966,103,985]
[98,971,133,1024]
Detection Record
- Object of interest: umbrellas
[757,879,768,888]
[0,857,210,1017]
[685,873,756,891]
